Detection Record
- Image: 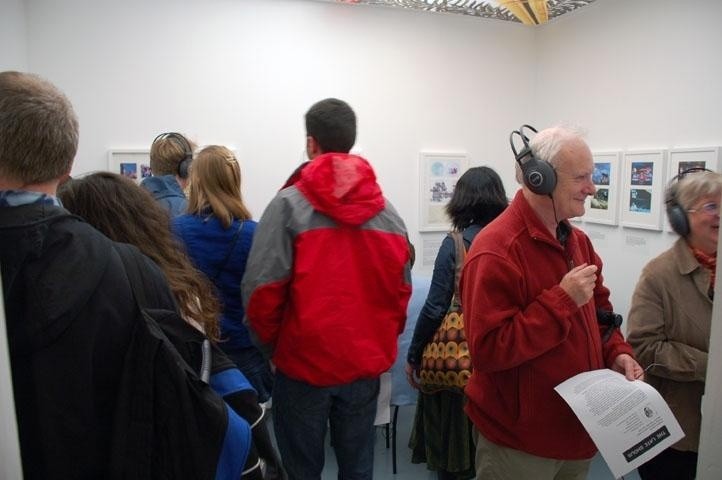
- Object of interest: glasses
[684,203,720,217]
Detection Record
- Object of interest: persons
[2,70,257,480]
[137,133,192,218]
[240,97,416,479]
[461,128,645,480]
[628,170,721,479]
[57,171,290,480]
[172,145,270,397]
[406,166,522,479]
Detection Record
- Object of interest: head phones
[666,167,713,234]
[510,125,557,195]
[153,133,192,177]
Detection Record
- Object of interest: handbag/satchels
[110,242,289,480]
[418,301,474,394]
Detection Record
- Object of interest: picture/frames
[109,150,154,184]
[569,150,619,225]
[666,147,722,232]
[417,150,468,232]
[622,150,666,232]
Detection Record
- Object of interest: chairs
[389,276,432,473]
